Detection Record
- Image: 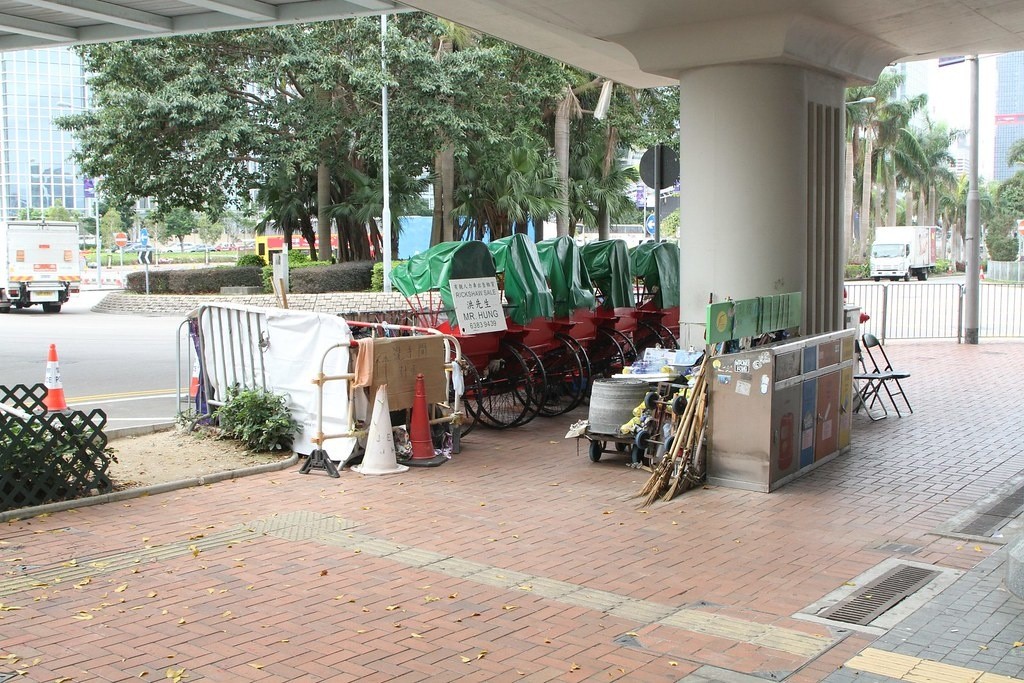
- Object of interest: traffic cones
[179,352,205,402]
[351,382,412,475]
[979,265,985,279]
[399,374,450,468]
[27,343,76,418]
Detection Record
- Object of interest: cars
[109,240,256,253]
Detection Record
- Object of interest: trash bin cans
[238,246,255,256]
[107,255,112,267]
[137,251,152,264]
[272,253,289,294]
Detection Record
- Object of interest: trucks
[1,220,82,314]
[869,226,938,280]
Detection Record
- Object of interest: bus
[574,223,644,248]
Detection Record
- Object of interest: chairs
[853,333,913,419]
[853,339,891,421]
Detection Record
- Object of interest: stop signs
[1018,220,1024,237]
[114,233,129,249]
